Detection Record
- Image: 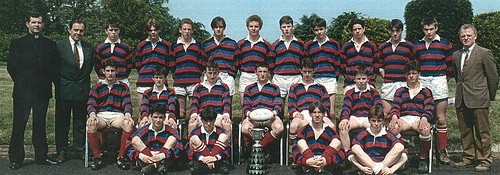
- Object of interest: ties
[74,41,80,69]
[461,50,469,71]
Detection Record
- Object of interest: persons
[95,15,304,118]
[291,100,346,175]
[412,17,454,164]
[339,19,380,92]
[287,57,336,144]
[338,65,382,152]
[241,62,285,147]
[304,18,341,115]
[139,65,178,131]
[52,19,95,162]
[187,60,232,142]
[124,104,183,175]
[376,19,414,106]
[346,106,409,175]
[450,23,499,171]
[184,106,231,175]
[86,59,134,170]
[6,12,58,169]
[388,60,435,173]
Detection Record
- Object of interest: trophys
[246,127,270,175]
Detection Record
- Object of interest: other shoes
[117,158,129,169]
[304,167,315,175]
[141,164,158,175]
[191,163,206,175]
[157,164,166,175]
[218,162,229,174]
[317,169,333,175]
[418,158,428,171]
[91,158,104,170]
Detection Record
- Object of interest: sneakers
[475,163,490,170]
[440,148,450,163]
[454,160,472,167]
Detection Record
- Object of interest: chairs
[400,123,440,173]
[84,127,121,168]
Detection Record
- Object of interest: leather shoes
[76,153,91,160]
[10,162,20,169]
[36,159,57,165]
[57,153,65,163]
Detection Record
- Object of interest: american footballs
[250,108,274,120]
